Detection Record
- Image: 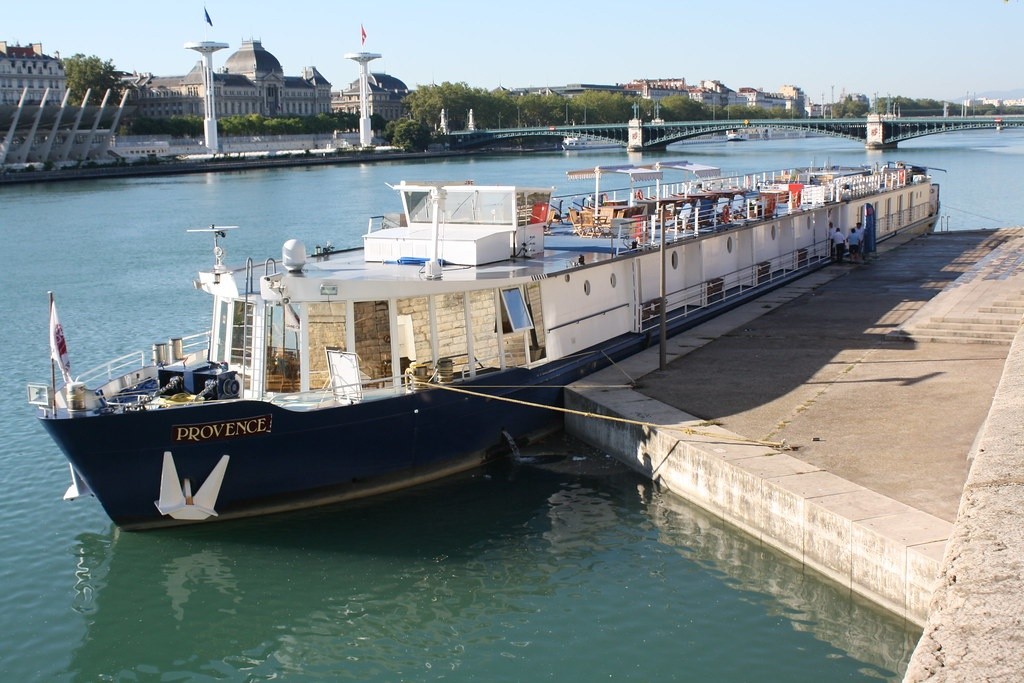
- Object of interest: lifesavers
[723,204,730,224]
[636,189,643,200]
[795,191,802,207]
[601,193,608,203]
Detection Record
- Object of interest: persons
[721,205,730,223]
[828,221,865,266]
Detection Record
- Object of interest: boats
[726,129,750,141]
[996,124,1003,130]
[562,133,622,149]
[26,161,940,531]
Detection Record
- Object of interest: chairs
[549,179,775,240]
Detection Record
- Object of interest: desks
[662,197,690,218]
[590,205,631,224]
[673,193,717,201]
[702,190,745,198]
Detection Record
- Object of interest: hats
[856,222,862,225]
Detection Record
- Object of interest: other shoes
[849,260,854,264]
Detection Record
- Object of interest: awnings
[566,164,663,235]
[652,161,721,219]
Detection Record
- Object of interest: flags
[203,4,214,27]
[49,300,71,384]
[360,22,368,47]
